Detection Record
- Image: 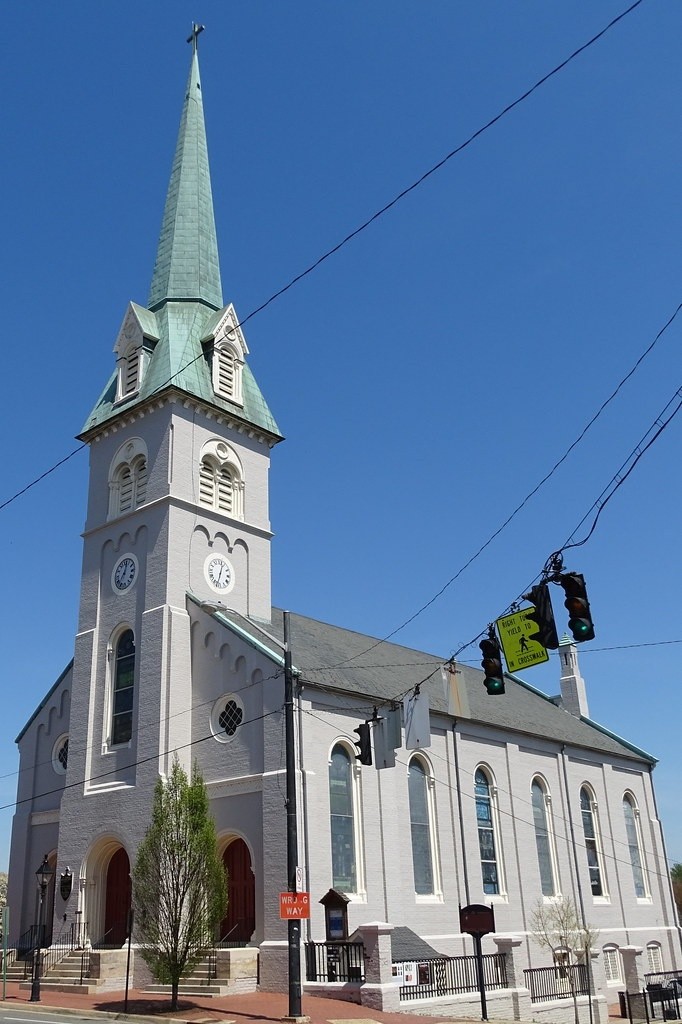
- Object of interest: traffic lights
[560,573,596,641]
[353,723,372,766]
[521,584,560,651]
[478,637,506,696]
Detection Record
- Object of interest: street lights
[28,853,54,1002]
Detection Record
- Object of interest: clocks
[112,553,140,595]
[204,552,235,596]
